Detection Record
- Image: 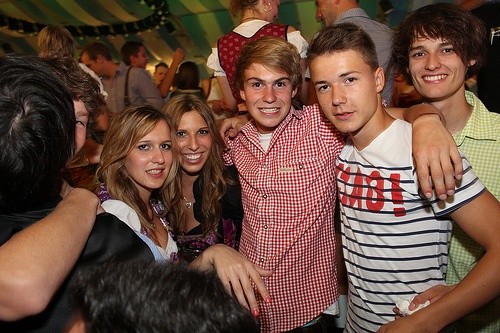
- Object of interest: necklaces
[240,17,267,22]
[183,196,194,208]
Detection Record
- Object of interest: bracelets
[412,112,442,125]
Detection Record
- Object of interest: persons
[391,1,500,333]
[207,0,311,115]
[222,35,463,333]
[0,49,273,333]
[220,23,500,333]
[81,41,165,112]
[305,0,395,107]
[38,23,109,131]
